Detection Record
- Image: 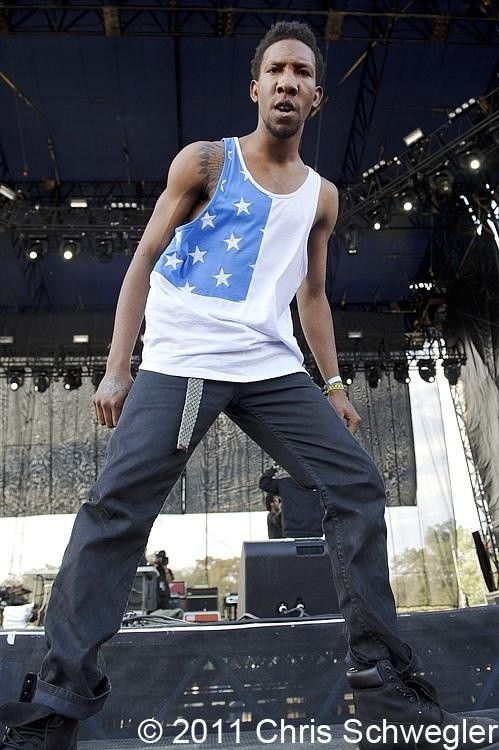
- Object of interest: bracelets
[321,375,347,396]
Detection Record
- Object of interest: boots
[0,672,84,750]
[346,663,498,750]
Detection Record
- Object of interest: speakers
[240,537,344,619]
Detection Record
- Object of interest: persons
[258,462,325,539]
[1,19,498,750]
[138,549,175,611]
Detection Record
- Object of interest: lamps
[338,143,486,256]
[5,360,139,393]
[335,356,464,389]
[16,230,147,264]
